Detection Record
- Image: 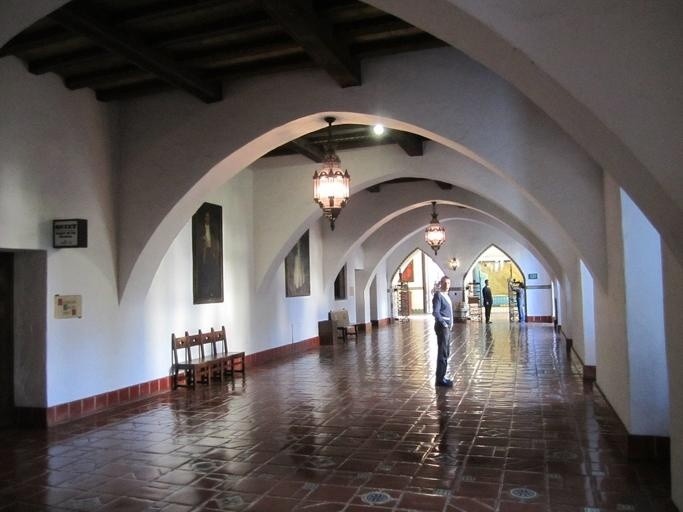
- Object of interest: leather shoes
[436,379,453,386]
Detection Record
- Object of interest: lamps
[452,257,458,271]
[425,202,445,256]
[313,116,350,232]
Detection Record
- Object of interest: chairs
[170,326,245,391]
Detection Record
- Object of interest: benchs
[329,308,358,343]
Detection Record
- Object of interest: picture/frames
[192,202,224,306]
[285,228,309,298]
[334,262,348,300]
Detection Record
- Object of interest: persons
[432,276,454,386]
[482,279,493,323]
[510,281,525,324]
[193,208,220,300]
